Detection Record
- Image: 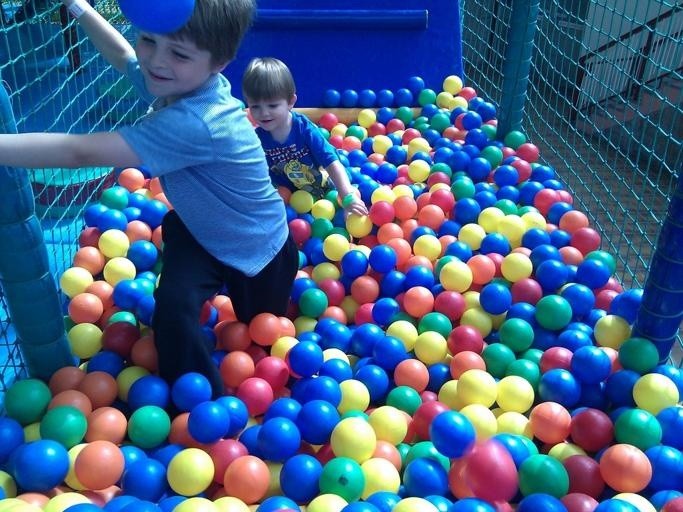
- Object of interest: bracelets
[67,0,89,19]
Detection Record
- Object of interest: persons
[0,0,297,408]
[242,55,371,217]
